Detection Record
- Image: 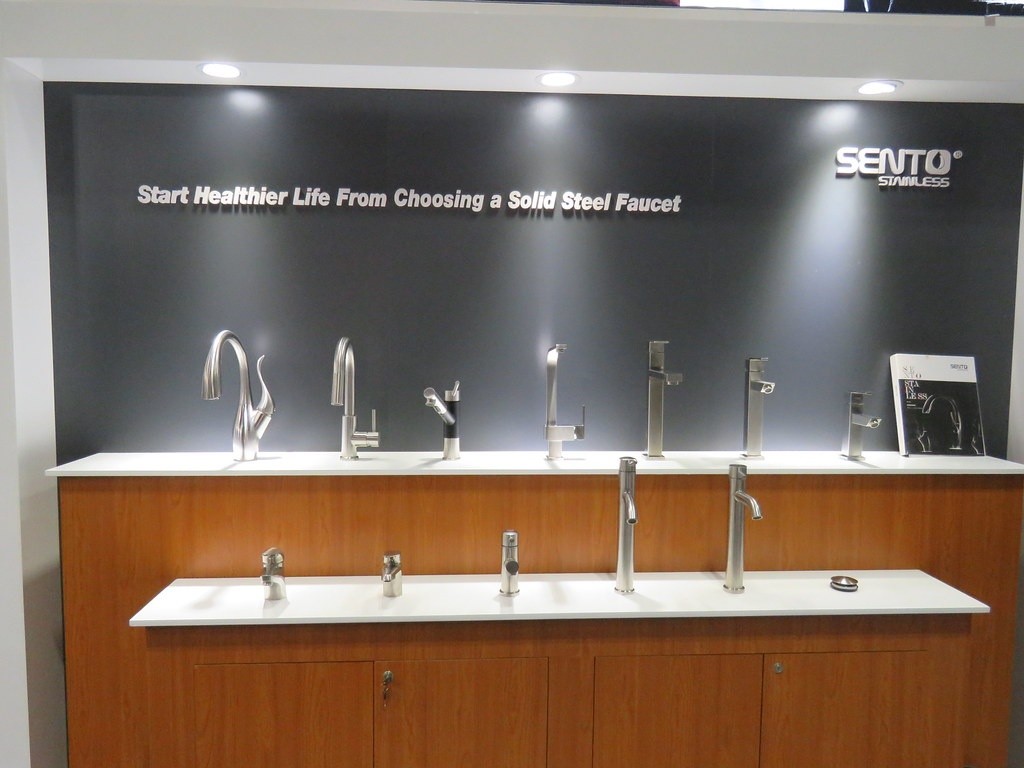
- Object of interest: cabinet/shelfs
[145,615,971,768]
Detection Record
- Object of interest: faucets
[331,338,380,459]
[202,330,274,462]
[616,457,637,592]
[647,339,684,456]
[422,380,460,459]
[501,529,519,595]
[261,547,285,600]
[723,464,762,594]
[382,551,401,596]
[544,344,585,458]
[744,356,775,456]
[841,391,882,457]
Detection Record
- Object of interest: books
[889,354,986,457]
